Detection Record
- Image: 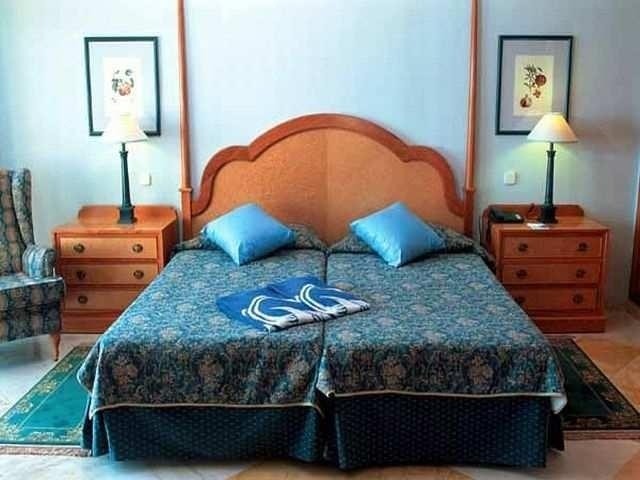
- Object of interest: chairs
[0,168,66,362]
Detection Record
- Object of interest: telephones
[487,207,524,223]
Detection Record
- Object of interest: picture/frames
[83,35,162,139]
[495,34,574,136]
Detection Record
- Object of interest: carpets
[0,341,95,459]
[544,335,640,441]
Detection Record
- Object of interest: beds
[96,113,560,467]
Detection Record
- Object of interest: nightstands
[51,203,180,336]
[482,204,611,333]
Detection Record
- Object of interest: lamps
[528,112,580,224]
[99,111,150,226]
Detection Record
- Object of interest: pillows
[348,201,447,269]
[202,205,295,268]
